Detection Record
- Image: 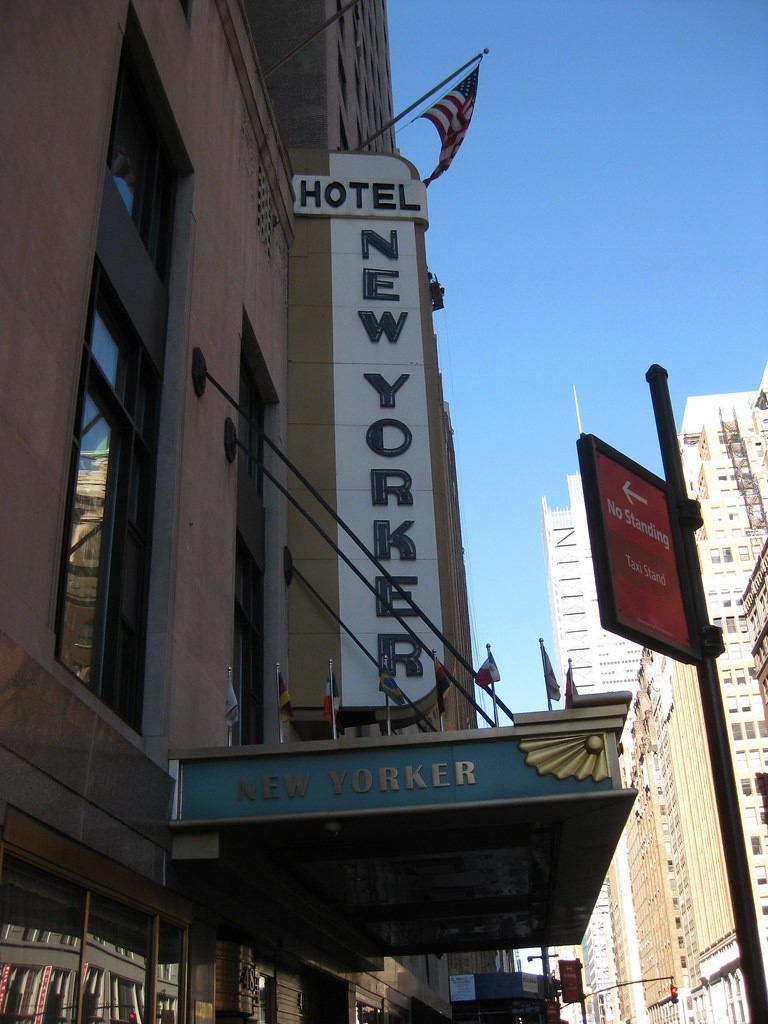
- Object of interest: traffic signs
[575,433,705,664]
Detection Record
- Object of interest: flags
[435,657,450,714]
[475,653,500,688]
[323,671,340,726]
[277,671,293,723]
[420,63,480,188]
[225,675,239,727]
[541,645,561,701]
[565,667,579,710]
[378,664,405,708]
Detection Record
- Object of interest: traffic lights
[551,978,561,998]
[670,985,678,1004]
[129,1012,137,1024]
